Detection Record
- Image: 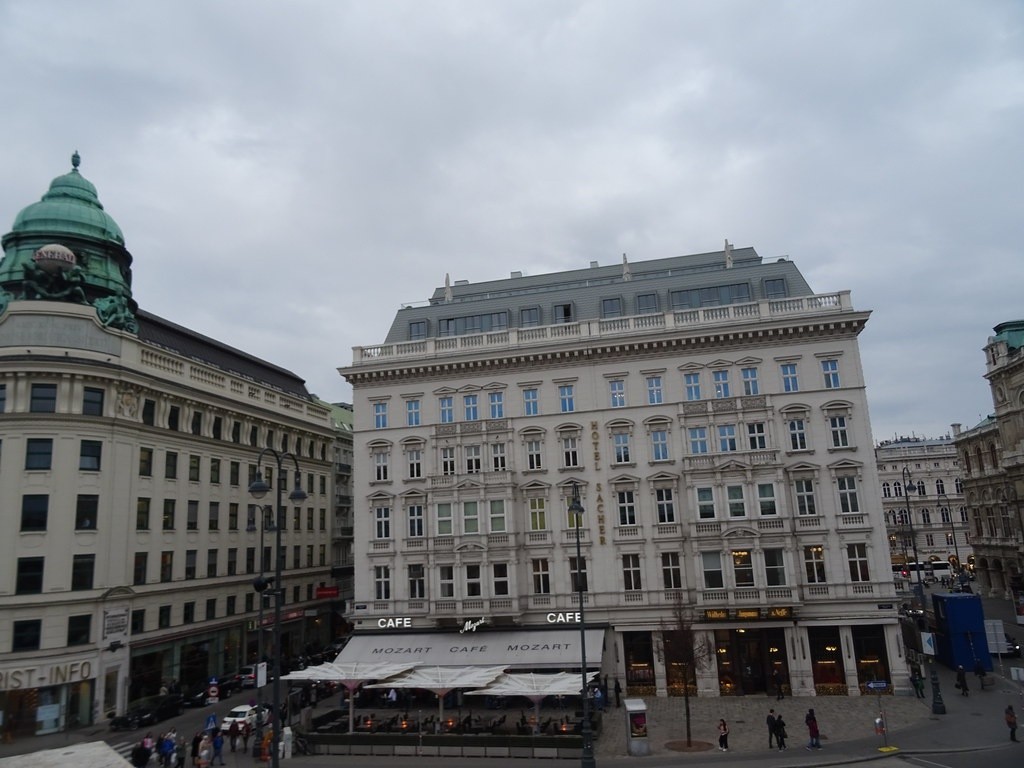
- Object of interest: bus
[892,561,952,584]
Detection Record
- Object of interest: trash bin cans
[874,717,885,736]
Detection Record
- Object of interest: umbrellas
[364,664,511,720]
[279,661,422,734]
[462,670,598,721]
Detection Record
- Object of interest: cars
[110,695,186,732]
[221,703,274,737]
[185,633,352,707]
[992,632,1021,659]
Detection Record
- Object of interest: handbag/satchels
[784,731,788,738]
[955,682,961,689]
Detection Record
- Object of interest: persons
[613,676,623,708]
[805,709,823,751]
[910,673,925,698]
[940,574,956,588]
[170,679,178,694]
[717,719,729,752]
[160,682,168,698]
[236,676,246,692]
[766,709,779,748]
[957,665,969,697]
[773,668,784,700]
[776,715,788,752]
[130,703,288,768]
[1005,705,1020,741]
[974,662,987,689]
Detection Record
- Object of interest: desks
[318,714,584,733]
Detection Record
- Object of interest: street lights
[246,502,276,682]
[568,481,597,768]
[902,467,946,715]
[247,448,308,768]
[935,493,963,594]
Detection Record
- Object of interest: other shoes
[719,747,723,750]
[723,749,728,751]
[785,746,788,750]
[806,747,812,751]
[818,748,822,750]
[779,750,784,752]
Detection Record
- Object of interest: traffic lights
[902,570,908,577]
